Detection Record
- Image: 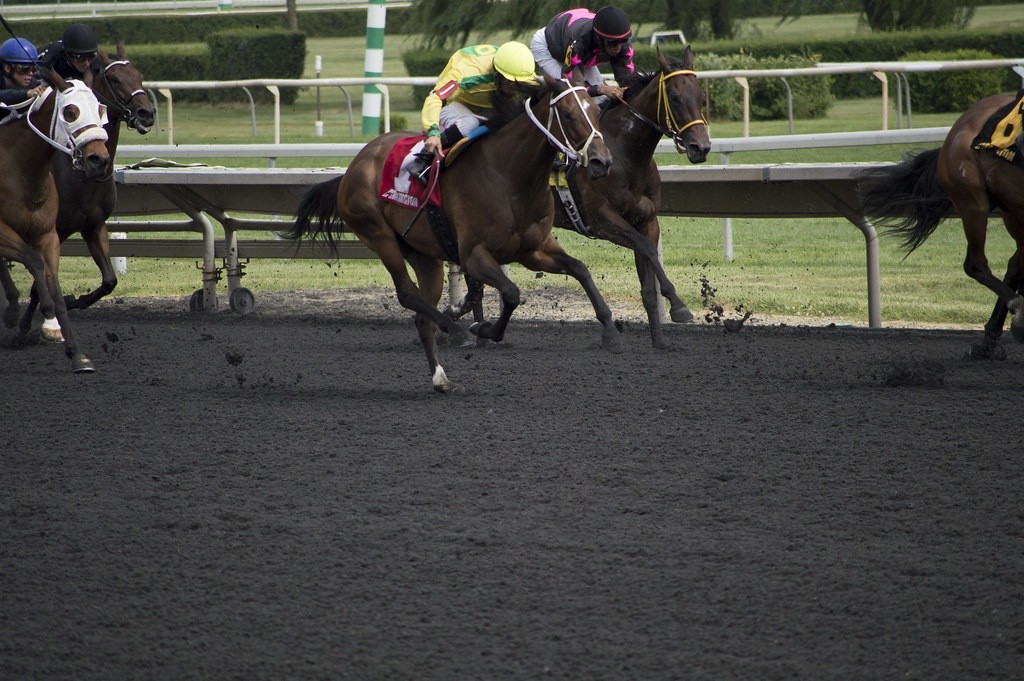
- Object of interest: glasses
[69,53,97,63]
[9,64,39,73]
[599,35,630,45]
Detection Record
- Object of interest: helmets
[63,24,97,54]
[0,37,43,63]
[493,41,536,83]
[592,7,633,41]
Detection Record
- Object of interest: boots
[407,125,464,186]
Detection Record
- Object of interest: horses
[844,90,1024,362]
[443,43,712,354]
[0,39,158,376]
[275,67,624,395]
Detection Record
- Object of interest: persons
[421,5,640,159]
[0,24,108,105]
